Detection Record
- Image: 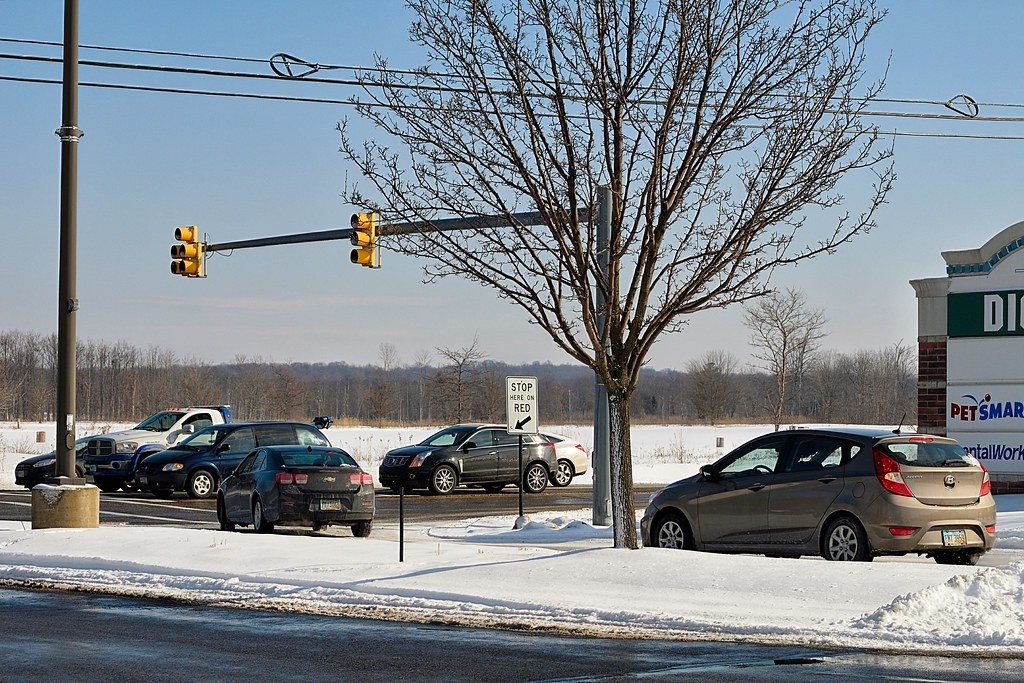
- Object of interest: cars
[464,421,591,489]
[14,434,106,492]
[215,445,375,538]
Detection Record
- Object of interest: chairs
[792,443,823,471]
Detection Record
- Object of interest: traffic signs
[505,375,538,435]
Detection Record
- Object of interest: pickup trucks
[82,405,329,494]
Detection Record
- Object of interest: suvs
[134,420,333,500]
[639,425,998,566]
[378,422,559,495]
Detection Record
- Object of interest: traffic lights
[170,242,203,276]
[350,210,375,268]
[175,226,197,243]
